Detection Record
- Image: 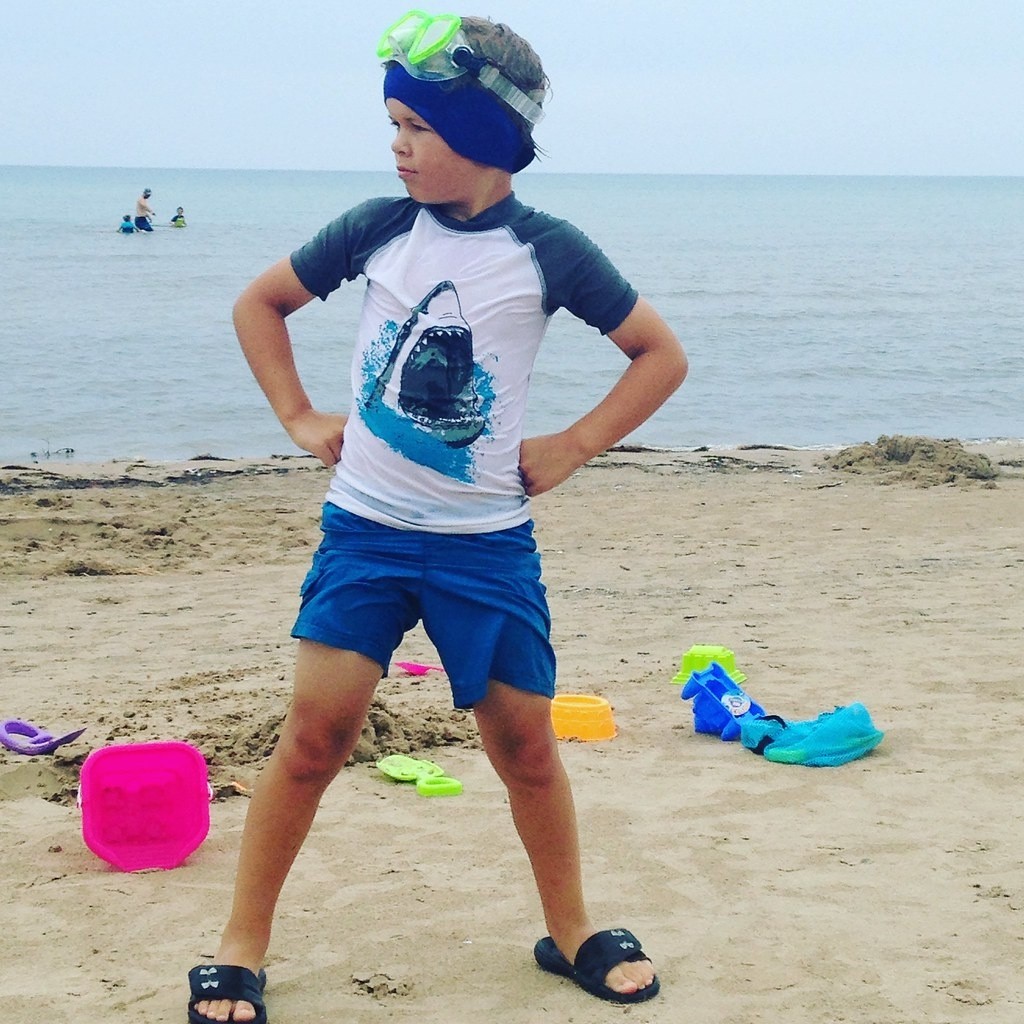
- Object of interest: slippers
[533,928,661,1004]
[188,964,267,1024]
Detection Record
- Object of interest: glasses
[377,9,544,125]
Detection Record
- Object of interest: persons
[135,189,155,232]
[170,207,187,227]
[188,19,688,1024]
[117,215,139,233]
